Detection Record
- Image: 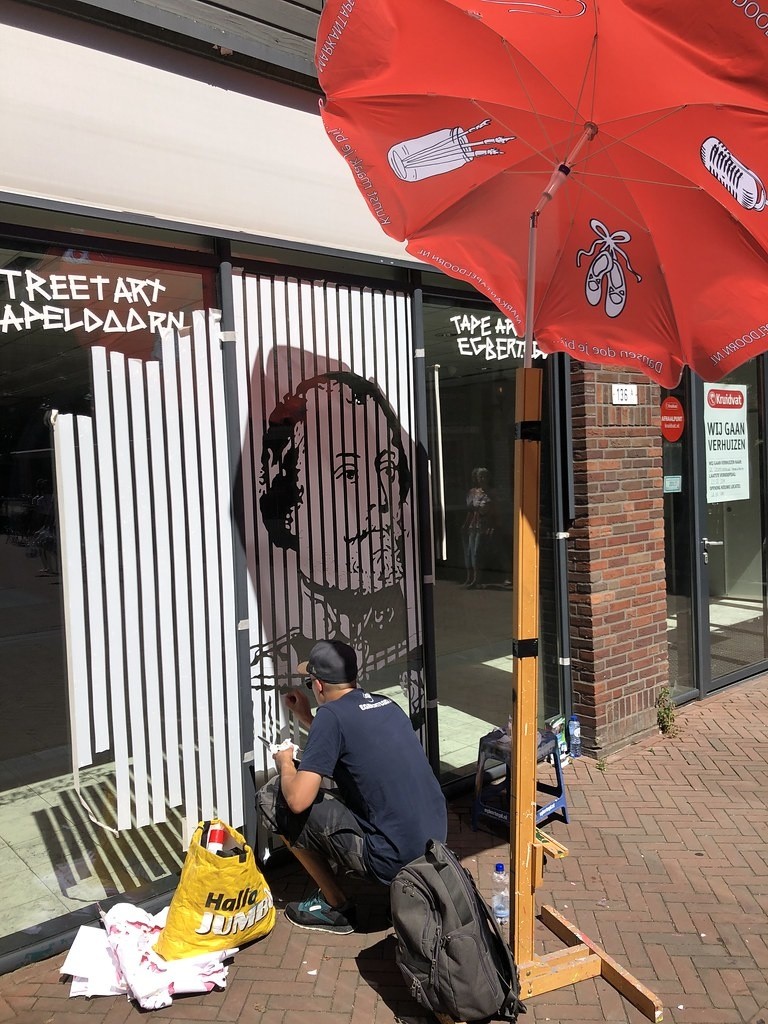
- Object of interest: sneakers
[285,886,359,935]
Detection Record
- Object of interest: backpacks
[389,837,528,1024]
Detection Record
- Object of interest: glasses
[305,677,317,689]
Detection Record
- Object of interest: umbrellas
[315,0,768,367]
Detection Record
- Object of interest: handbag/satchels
[150,820,276,963]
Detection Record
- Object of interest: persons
[460,466,493,588]
[257,640,448,935]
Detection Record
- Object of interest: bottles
[491,863,510,926]
[569,716,581,757]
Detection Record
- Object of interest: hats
[296,639,358,685]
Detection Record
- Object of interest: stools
[471,727,570,827]
